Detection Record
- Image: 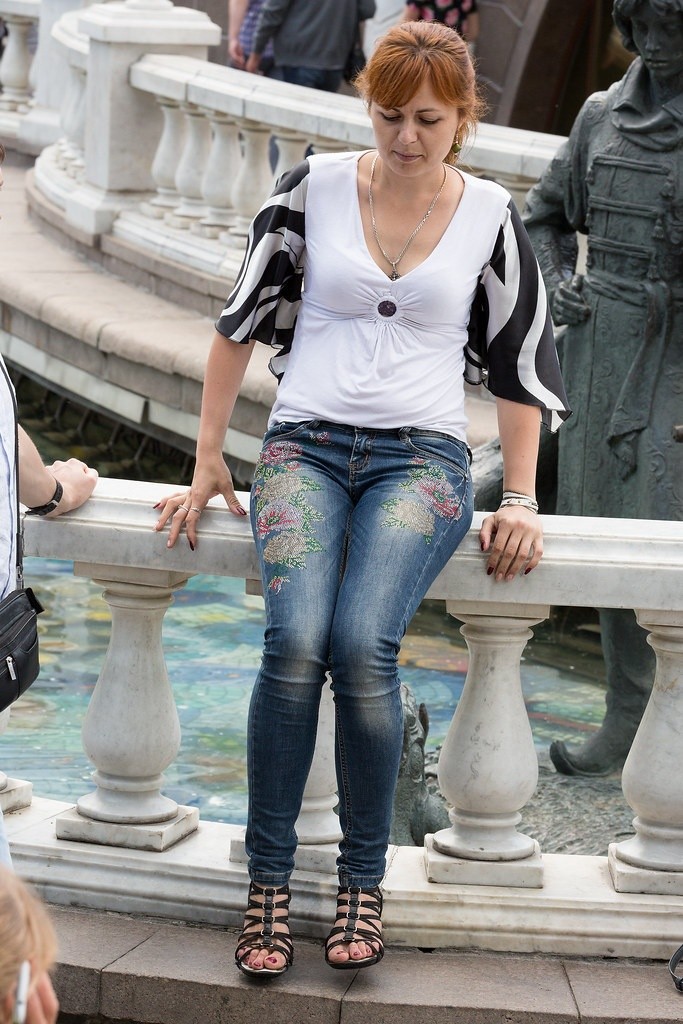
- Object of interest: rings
[190,508,201,514]
[178,504,189,511]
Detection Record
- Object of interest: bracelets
[501,491,538,514]
[25,477,63,517]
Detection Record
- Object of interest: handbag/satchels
[0,587,45,712]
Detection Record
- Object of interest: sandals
[325,886,384,969]
[235,880,293,977]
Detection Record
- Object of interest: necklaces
[369,154,447,282]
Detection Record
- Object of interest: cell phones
[13,960,30,1024]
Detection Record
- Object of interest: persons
[521,0,683,780]
[153,20,573,979]
[0,865,60,1024]
[228,0,479,174]
[0,355,99,873]
